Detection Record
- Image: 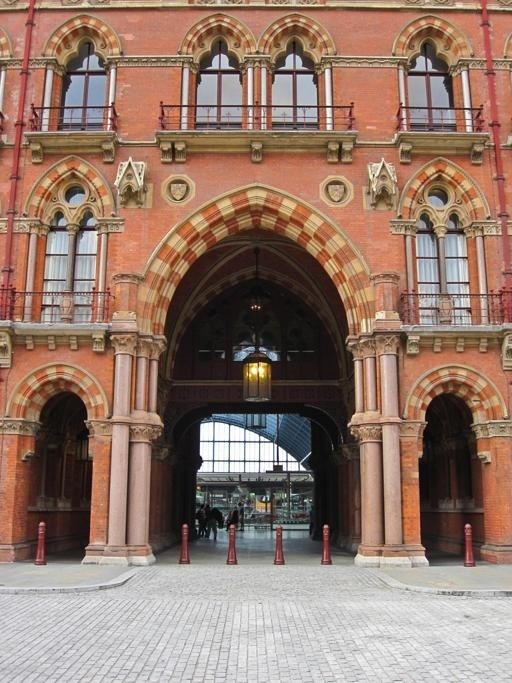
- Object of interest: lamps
[241,246,274,402]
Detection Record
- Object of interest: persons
[196,502,245,541]
